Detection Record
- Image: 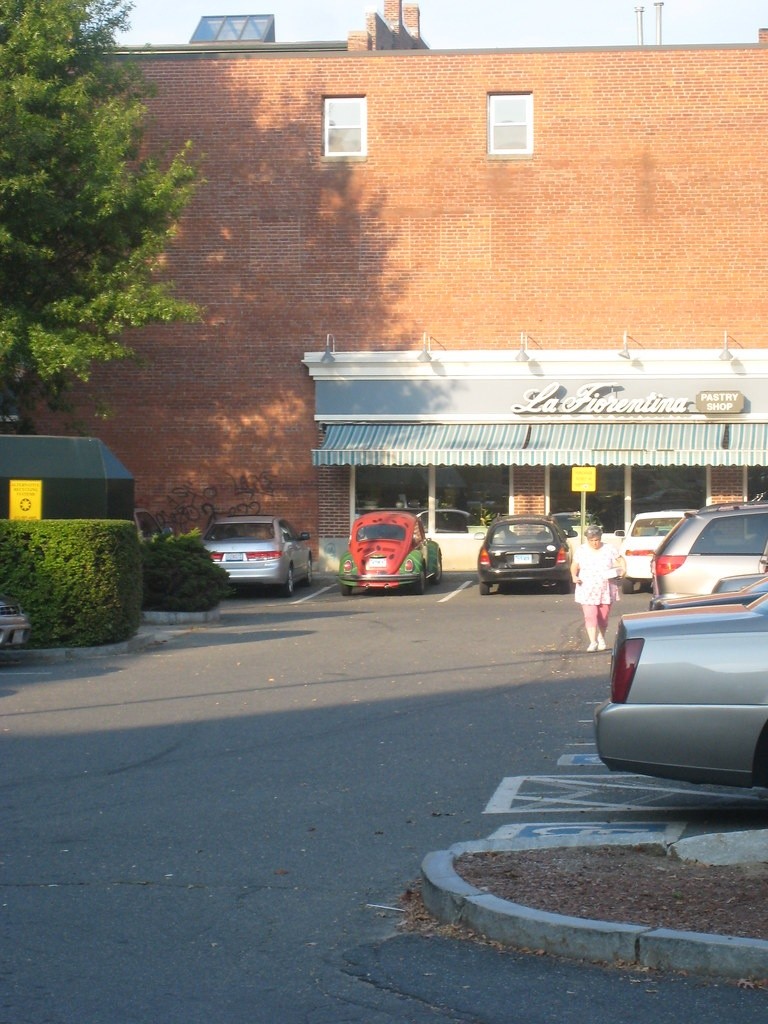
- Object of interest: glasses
[587,529,599,534]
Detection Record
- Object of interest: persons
[570,526,626,651]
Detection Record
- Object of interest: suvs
[647,501,768,599]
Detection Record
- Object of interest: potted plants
[570,511,602,538]
[465,507,496,535]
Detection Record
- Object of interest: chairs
[224,527,238,537]
[713,523,744,545]
[536,531,551,540]
[639,526,657,535]
[498,529,515,538]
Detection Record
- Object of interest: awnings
[310,424,768,467]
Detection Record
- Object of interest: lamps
[416,332,433,362]
[617,330,630,359]
[319,334,335,363]
[718,331,733,361]
[513,332,529,362]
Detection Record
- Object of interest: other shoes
[597,634,607,651]
[587,641,597,652]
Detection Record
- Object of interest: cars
[478,514,579,596]
[648,571,768,612]
[133,508,174,544]
[614,507,699,593]
[594,593,768,790]
[337,512,443,595]
[202,514,314,598]
[415,508,475,534]
[0,593,32,649]
